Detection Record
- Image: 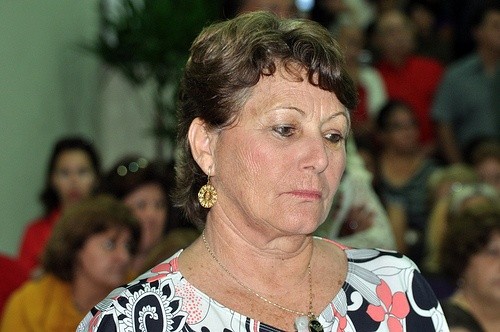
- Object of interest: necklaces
[201,229,325,332]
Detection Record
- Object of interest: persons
[0,126,205,332]
[73,10,449,332]
[243,1,499,332]
